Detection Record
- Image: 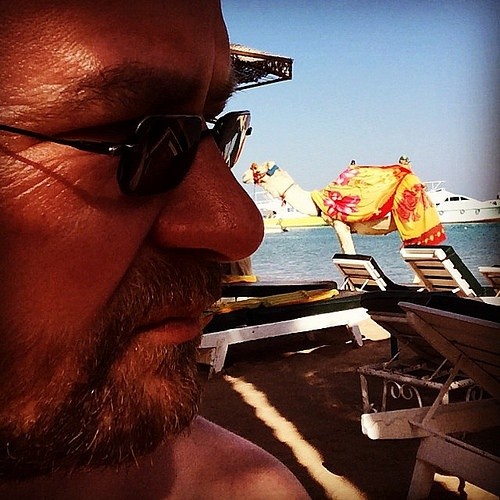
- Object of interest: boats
[436,194,500,226]
[249,180,454,231]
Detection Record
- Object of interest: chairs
[332,244,499,500]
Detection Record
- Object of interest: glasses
[0,111,252,195]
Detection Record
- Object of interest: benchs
[194,273,368,383]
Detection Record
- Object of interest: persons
[0,0,313,500]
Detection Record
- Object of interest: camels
[242,156,447,292]
